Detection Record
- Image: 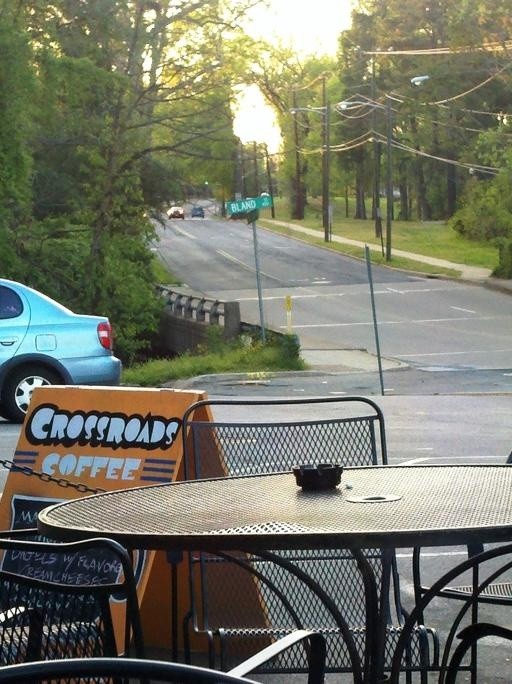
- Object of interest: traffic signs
[227,195,273,215]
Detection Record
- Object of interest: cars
[167,206,185,219]
[0,277,121,422]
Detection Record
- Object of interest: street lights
[336,100,393,262]
[411,68,487,86]
[288,105,330,242]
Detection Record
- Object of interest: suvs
[191,206,204,218]
